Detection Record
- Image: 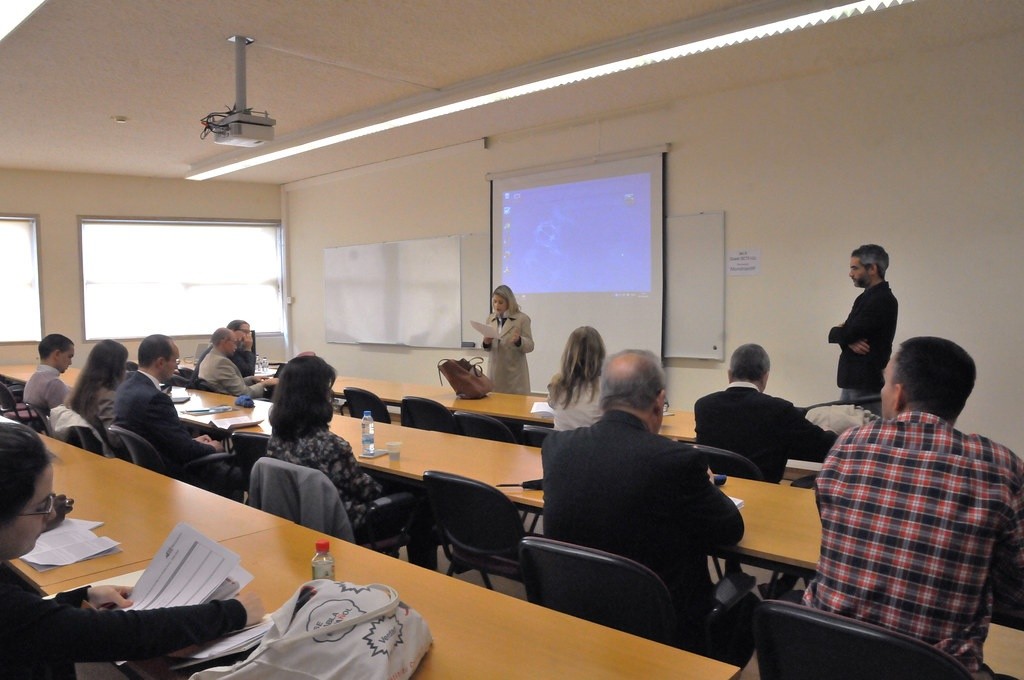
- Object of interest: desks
[170,386,823,579]
[1,415,294,589]
[40,523,742,680]
[331,377,824,471]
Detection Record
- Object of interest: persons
[695,344,839,484]
[115,334,229,493]
[189,320,255,393]
[828,243,898,417]
[22,333,75,410]
[266,356,437,572]
[541,350,759,670]
[482,285,535,393]
[198,328,279,399]
[547,325,607,431]
[62,340,128,449]
[0,422,264,680]
[802,335,1024,680]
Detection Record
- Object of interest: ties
[497,318,502,335]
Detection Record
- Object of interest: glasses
[168,358,181,364]
[664,396,669,413]
[19,495,54,522]
[241,328,253,336]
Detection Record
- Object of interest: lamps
[182,0,917,181]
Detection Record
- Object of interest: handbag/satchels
[438,357,493,398]
[184,577,433,677]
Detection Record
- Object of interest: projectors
[216,123,274,146]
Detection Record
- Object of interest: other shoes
[758,581,777,600]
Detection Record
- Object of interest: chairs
[0,360,975,680]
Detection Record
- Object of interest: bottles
[255,354,269,373]
[311,540,336,582]
[360,410,375,457]
